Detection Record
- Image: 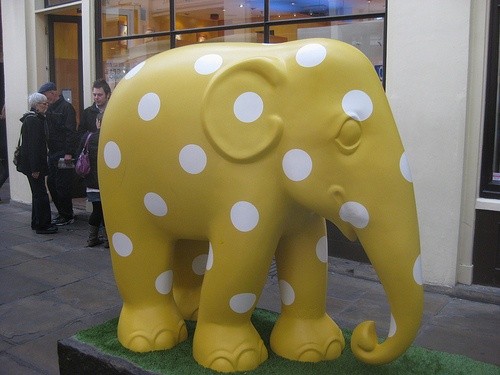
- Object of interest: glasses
[38,102,49,105]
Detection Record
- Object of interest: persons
[37,82,77,225]
[75,109,112,248]
[76,79,112,146]
[13,93,59,234]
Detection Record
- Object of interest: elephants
[97,37,424,373]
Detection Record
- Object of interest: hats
[39,83,56,93]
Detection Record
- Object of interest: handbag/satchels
[13,115,36,165]
[75,132,92,177]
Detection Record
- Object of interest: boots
[88,224,102,247]
[100,226,108,248]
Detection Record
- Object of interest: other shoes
[36,226,58,234]
[51,217,75,226]
